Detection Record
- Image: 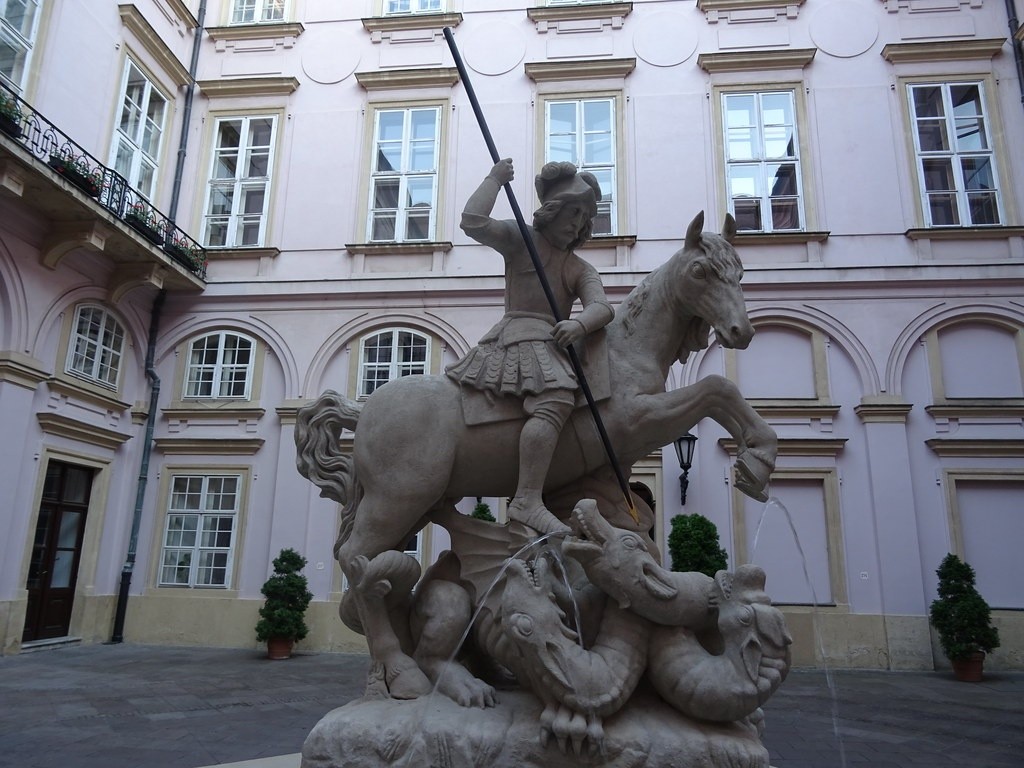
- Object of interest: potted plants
[0,89,22,139]
[927,551,1001,683]
[256,547,311,660]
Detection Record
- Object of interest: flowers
[57,150,110,194]
[130,205,163,235]
[162,226,209,267]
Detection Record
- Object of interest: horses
[290,209,781,699]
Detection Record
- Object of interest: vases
[49,156,100,197]
[165,244,197,272]
[126,214,163,245]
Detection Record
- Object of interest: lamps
[674,431,698,506]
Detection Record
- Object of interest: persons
[444,158,615,538]
